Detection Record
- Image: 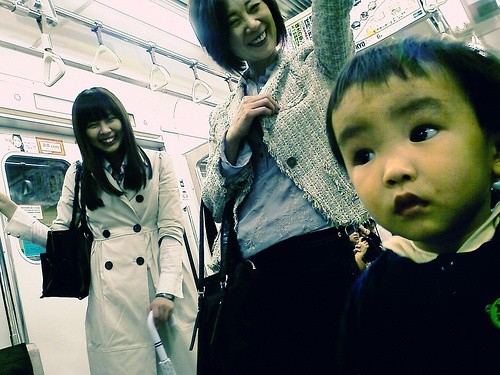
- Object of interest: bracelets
[155,293,175,301]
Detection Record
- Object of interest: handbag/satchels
[190,199,275,375]
[40,165,94,299]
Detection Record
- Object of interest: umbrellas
[147,308,177,375]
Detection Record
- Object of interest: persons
[188,0,376,375]
[326,36,500,375]
[0,86,199,375]
